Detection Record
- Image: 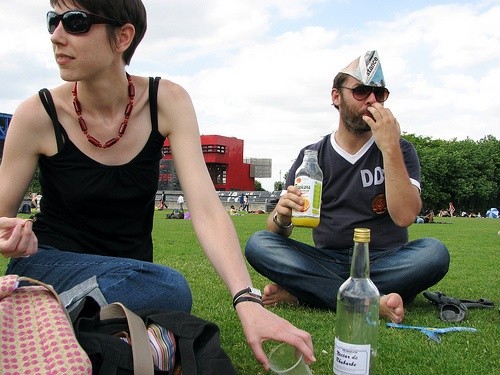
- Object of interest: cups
[268,343,312,375]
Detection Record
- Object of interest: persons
[0,0,316,375]
[229,193,251,216]
[416,200,481,223]
[244,50,450,325]
[177,194,186,208]
[159,191,168,210]
[170,208,184,219]
[21,191,44,215]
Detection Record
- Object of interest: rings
[21,252,31,258]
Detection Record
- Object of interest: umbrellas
[485,208,499,219]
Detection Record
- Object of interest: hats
[340,50,386,87]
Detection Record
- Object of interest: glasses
[46,10,126,34]
[338,86,389,103]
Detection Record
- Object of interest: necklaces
[72,71,135,148]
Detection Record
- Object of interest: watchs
[272,211,293,229]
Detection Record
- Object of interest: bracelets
[233,286,264,310]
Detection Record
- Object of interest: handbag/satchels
[0,275,93,375]
[69,303,237,375]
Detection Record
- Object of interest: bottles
[331,227,380,375]
[291,150,323,227]
